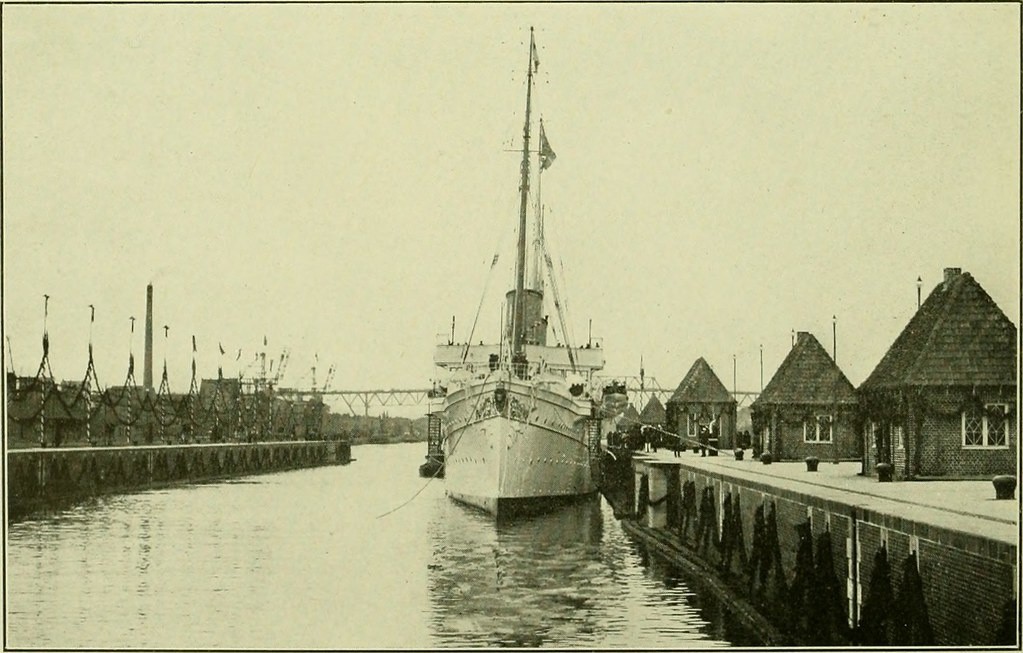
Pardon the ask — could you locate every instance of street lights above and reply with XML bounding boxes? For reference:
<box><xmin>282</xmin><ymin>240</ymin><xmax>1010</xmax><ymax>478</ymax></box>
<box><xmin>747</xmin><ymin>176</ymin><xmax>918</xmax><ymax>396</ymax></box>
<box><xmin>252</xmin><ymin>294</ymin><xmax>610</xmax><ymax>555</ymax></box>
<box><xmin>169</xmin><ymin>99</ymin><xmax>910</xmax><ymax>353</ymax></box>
<box><xmin>917</xmin><ymin>275</ymin><xmax>923</xmax><ymax>309</ymax></box>
<box><xmin>832</xmin><ymin>314</ymin><xmax>837</xmax><ymax>363</ymax></box>
<box><xmin>759</xmin><ymin>344</ymin><xmax>764</xmax><ymax>392</ymax></box>
<box><xmin>733</xmin><ymin>354</ymin><xmax>737</xmax><ymax>400</ymax></box>
<box><xmin>791</xmin><ymin>328</ymin><xmax>795</xmax><ymax>347</ymax></box>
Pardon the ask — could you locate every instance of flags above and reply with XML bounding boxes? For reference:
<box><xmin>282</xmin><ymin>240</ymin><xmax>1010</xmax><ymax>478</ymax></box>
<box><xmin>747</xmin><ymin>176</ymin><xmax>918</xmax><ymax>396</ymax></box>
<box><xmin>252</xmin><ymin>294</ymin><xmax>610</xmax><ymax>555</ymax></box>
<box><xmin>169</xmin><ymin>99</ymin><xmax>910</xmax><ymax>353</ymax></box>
<box><xmin>540</xmin><ymin>125</ymin><xmax>557</xmax><ymax>173</ymax></box>
<box><xmin>532</xmin><ymin>33</ymin><xmax>540</xmax><ymax>72</ymax></box>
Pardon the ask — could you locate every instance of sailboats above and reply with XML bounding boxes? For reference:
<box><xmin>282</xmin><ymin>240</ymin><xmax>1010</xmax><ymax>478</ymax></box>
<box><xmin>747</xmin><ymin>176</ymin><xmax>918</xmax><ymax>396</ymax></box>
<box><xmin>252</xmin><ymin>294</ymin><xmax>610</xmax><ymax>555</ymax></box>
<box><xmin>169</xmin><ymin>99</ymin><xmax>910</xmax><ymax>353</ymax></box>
<box><xmin>431</xmin><ymin>25</ymin><xmax>604</xmax><ymax>521</ymax></box>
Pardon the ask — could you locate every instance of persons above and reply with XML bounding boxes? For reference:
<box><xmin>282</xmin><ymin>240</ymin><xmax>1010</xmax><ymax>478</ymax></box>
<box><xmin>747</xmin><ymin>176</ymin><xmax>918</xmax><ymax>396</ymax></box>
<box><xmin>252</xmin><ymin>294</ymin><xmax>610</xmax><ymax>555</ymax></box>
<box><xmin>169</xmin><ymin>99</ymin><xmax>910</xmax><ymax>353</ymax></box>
<box><xmin>608</xmin><ymin>423</ymin><xmax>710</xmax><ymax>458</ymax></box>
